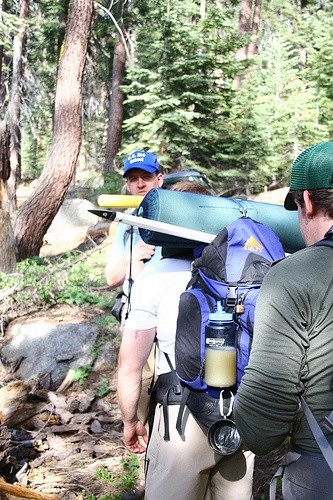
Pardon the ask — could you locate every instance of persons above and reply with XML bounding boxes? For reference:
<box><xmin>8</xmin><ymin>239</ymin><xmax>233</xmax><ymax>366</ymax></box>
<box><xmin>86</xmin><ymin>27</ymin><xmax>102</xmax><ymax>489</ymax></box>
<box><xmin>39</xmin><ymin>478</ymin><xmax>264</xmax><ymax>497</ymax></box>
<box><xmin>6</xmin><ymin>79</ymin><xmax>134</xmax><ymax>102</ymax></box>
<box><xmin>234</xmin><ymin>141</ymin><xmax>332</xmax><ymax>500</ymax></box>
<box><xmin>105</xmin><ymin>151</ymin><xmax>165</xmax><ymax>286</ymax></box>
<box><xmin>116</xmin><ymin>181</ymin><xmax>255</xmax><ymax>500</ymax></box>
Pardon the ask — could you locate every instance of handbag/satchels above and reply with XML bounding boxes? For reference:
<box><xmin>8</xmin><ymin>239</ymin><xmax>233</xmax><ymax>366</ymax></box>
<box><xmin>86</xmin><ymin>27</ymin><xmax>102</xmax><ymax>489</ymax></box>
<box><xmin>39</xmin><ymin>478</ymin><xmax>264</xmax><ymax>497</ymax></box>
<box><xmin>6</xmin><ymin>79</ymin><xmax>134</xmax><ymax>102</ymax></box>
<box><xmin>111</xmin><ymin>291</ymin><xmax>128</xmax><ymax>321</ymax></box>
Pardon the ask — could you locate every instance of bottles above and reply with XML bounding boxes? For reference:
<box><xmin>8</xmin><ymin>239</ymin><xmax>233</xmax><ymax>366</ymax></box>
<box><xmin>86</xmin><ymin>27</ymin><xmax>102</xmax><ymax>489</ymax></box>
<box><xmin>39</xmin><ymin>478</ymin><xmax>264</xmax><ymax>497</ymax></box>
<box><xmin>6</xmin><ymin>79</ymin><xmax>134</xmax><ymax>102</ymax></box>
<box><xmin>205</xmin><ymin>301</ymin><xmax>237</xmax><ymax>387</ymax></box>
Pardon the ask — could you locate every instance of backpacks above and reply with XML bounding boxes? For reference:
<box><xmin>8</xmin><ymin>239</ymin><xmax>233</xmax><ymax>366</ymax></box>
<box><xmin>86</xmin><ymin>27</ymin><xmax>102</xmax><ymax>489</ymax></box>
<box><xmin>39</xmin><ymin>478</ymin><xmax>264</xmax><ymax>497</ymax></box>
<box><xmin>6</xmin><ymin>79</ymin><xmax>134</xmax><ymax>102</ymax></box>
<box><xmin>145</xmin><ymin>216</ymin><xmax>285</xmax><ymax>441</ymax></box>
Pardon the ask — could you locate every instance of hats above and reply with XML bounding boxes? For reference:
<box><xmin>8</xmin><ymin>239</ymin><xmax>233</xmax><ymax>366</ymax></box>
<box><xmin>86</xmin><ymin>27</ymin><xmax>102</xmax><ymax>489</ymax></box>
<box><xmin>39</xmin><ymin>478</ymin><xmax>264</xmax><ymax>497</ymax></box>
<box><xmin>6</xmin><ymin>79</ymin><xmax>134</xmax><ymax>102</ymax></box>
<box><xmin>284</xmin><ymin>138</ymin><xmax>333</xmax><ymax>211</ymax></box>
<box><xmin>122</xmin><ymin>151</ymin><xmax>161</xmax><ymax>177</ymax></box>
